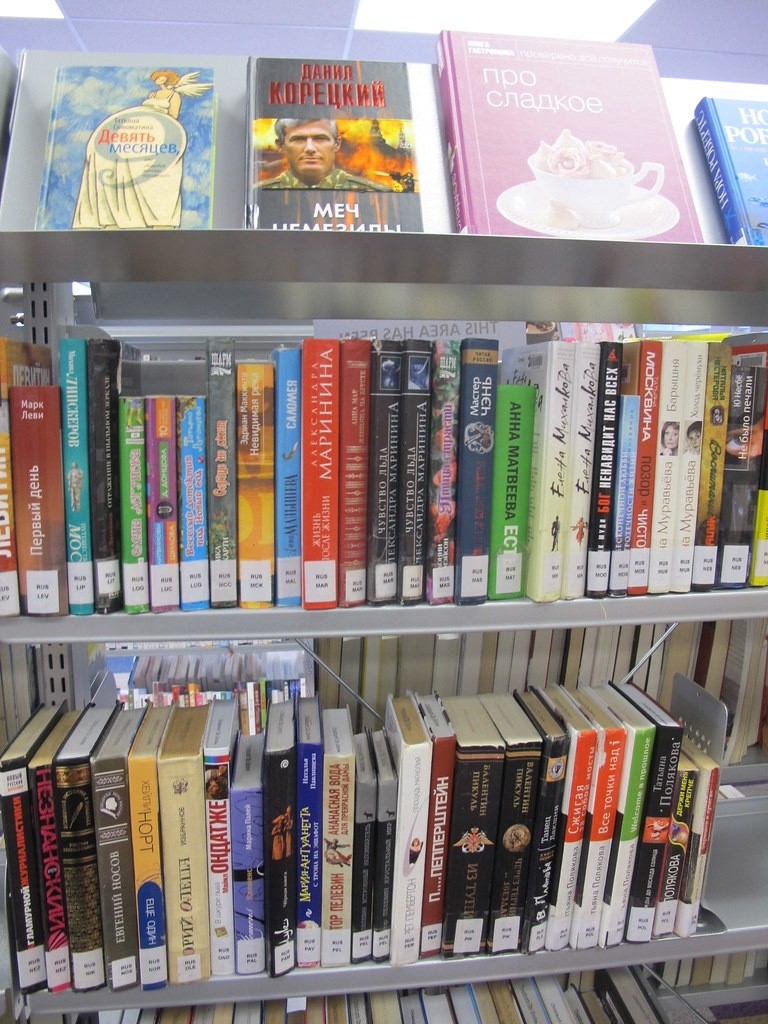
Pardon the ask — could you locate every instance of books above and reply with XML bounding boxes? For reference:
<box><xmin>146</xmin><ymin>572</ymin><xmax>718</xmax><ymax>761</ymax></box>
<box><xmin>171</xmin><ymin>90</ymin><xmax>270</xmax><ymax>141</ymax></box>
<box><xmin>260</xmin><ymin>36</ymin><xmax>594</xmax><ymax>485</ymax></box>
<box><xmin>0</xmin><ymin>616</ymin><xmax>768</xmax><ymax>1024</ymax></box>
<box><xmin>0</xmin><ymin>30</ymin><xmax>706</xmax><ymax>244</ymax></box>
<box><xmin>694</xmin><ymin>96</ymin><xmax>768</xmax><ymax>247</ymax></box>
<box><xmin>0</xmin><ymin>337</ymin><xmax>768</xmax><ymax>620</ymax></box>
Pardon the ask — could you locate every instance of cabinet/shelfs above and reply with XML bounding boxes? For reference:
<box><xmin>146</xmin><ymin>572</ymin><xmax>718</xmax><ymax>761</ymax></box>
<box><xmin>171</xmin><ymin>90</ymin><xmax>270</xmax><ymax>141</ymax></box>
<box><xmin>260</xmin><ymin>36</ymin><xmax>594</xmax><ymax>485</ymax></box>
<box><xmin>2</xmin><ymin>50</ymin><xmax>767</xmax><ymax>1023</ymax></box>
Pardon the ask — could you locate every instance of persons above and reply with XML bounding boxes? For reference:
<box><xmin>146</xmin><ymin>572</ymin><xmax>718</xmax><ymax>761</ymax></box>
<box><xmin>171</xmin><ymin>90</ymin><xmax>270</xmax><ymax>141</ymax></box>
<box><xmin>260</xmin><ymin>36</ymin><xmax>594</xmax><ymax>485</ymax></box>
<box><xmin>255</xmin><ymin>116</ymin><xmax>394</xmax><ymax>193</ymax></box>
<box><xmin>660</xmin><ymin>421</ymin><xmax>678</xmax><ymax>455</ymax></box>
<box><xmin>685</xmin><ymin>421</ymin><xmax>702</xmax><ymax>455</ymax></box>
<box><xmin>724</xmin><ymin>364</ymin><xmax>768</xmax><ymax>467</ymax></box>
